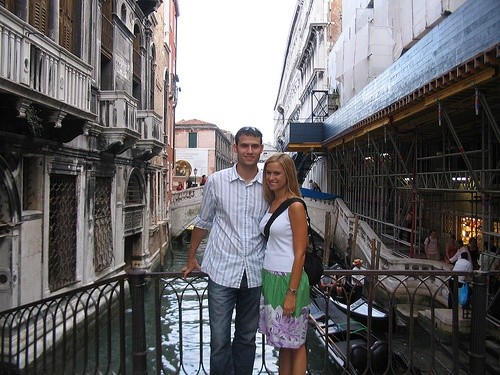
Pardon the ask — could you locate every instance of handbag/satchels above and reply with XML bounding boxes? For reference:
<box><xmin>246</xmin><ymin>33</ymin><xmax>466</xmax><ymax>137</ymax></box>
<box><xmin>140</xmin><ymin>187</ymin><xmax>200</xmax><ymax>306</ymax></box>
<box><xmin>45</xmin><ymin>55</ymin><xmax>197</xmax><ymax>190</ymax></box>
<box><xmin>304</xmin><ymin>252</ymin><xmax>324</xmax><ymax>285</ymax></box>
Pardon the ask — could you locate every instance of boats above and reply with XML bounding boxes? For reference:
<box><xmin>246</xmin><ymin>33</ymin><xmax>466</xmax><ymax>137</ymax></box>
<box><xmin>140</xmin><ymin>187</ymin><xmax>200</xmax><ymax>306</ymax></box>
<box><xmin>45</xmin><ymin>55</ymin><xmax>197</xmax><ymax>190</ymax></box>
<box><xmin>308</xmin><ymin>288</ymin><xmax>423</xmax><ymax>375</ymax></box>
<box><xmin>313</xmin><ymin>275</ymin><xmax>389</xmax><ymax>319</ymax></box>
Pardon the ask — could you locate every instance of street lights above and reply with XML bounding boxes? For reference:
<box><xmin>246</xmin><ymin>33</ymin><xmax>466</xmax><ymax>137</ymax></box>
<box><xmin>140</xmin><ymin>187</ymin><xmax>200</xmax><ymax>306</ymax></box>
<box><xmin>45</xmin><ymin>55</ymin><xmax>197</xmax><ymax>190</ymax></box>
<box><xmin>194</xmin><ymin>168</ymin><xmax>197</xmax><ymax>185</ymax></box>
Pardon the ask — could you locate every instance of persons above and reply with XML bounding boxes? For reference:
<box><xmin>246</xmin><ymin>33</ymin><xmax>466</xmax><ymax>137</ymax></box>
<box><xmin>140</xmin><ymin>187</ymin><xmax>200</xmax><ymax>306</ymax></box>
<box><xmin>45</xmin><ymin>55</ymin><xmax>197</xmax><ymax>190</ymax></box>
<box><xmin>331</xmin><ymin>259</ymin><xmax>368</xmax><ymax>305</ymax></box>
<box><xmin>200</xmin><ymin>175</ymin><xmax>207</xmax><ymax>186</ymax></box>
<box><xmin>424</xmin><ymin>230</ymin><xmax>441</xmax><ymax>260</ymax></box>
<box><xmin>258</xmin><ymin>153</ymin><xmax>325</xmax><ymax>375</ymax></box>
<box><xmin>186</xmin><ymin>177</ymin><xmax>193</xmax><ymax>189</ymax></box>
<box><xmin>445</xmin><ymin>234</ymin><xmax>480</xmax><ymax>310</ymax></box>
<box><xmin>176</xmin><ymin>181</ymin><xmax>184</xmax><ymax>191</ymax></box>
<box><xmin>181</xmin><ymin>127</ymin><xmax>269</xmax><ymax>375</ymax></box>
<box><xmin>309</xmin><ymin>180</ymin><xmax>321</xmax><ymax>192</ymax></box>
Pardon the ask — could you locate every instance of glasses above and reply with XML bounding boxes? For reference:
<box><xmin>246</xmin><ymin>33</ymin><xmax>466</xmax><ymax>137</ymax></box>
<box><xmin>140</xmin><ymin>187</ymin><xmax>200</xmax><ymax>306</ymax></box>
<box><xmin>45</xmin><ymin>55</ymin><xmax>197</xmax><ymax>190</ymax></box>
<box><xmin>237</xmin><ymin>126</ymin><xmax>262</xmax><ymax>137</ymax></box>
